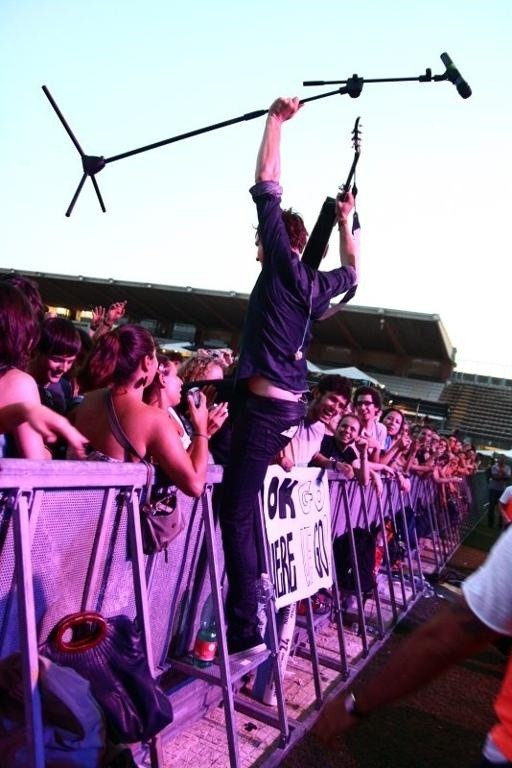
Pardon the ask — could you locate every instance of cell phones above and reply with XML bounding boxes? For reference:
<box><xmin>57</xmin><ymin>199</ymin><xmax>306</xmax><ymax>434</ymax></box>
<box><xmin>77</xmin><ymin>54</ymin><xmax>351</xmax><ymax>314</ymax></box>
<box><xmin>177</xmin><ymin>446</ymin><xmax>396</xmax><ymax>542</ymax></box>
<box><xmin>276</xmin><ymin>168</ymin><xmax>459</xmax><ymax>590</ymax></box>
<box><xmin>191</xmin><ymin>387</ymin><xmax>200</xmax><ymax>408</ymax></box>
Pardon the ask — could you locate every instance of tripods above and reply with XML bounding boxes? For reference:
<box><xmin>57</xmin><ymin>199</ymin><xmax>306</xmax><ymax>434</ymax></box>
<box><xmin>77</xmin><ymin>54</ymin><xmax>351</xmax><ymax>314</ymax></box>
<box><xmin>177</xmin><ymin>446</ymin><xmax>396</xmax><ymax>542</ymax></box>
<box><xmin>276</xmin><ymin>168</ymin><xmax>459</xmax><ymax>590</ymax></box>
<box><xmin>41</xmin><ymin>73</ymin><xmax>441</xmax><ymax>218</ymax></box>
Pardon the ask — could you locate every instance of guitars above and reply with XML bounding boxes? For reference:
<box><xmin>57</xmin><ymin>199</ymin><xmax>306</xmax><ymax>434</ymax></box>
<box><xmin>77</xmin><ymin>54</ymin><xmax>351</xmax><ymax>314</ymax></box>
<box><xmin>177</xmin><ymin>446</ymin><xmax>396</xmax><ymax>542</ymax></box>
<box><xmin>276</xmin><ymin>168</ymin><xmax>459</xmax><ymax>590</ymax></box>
<box><xmin>299</xmin><ymin>117</ymin><xmax>362</xmax><ymax>269</ymax></box>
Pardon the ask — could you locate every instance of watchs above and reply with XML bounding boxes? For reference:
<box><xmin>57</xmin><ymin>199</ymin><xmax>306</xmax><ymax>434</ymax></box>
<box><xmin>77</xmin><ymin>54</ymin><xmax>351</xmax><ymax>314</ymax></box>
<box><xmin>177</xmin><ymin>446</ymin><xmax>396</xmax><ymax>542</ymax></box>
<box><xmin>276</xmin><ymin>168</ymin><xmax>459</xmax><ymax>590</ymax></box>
<box><xmin>344</xmin><ymin>691</ymin><xmax>372</xmax><ymax>722</ymax></box>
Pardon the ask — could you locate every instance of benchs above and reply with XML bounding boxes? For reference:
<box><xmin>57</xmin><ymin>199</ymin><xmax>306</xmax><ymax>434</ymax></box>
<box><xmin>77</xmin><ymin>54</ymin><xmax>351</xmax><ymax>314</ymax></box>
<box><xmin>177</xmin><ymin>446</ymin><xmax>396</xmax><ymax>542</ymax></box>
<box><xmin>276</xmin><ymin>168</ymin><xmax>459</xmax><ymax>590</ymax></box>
<box><xmin>365</xmin><ymin>372</ymin><xmax>512</xmax><ymax>450</ymax></box>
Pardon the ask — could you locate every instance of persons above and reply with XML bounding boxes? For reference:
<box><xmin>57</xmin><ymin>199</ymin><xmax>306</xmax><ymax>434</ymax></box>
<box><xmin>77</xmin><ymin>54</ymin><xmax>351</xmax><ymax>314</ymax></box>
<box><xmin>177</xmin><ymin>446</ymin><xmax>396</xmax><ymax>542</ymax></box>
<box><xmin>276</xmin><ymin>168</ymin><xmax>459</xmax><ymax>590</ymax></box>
<box><xmin>310</xmin><ymin>455</ymin><xmax>511</xmax><ymax>768</ymax></box>
<box><xmin>216</xmin><ymin>96</ymin><xmax>361</xmax><ymax>664</ymax></box>
<box><xmin>463</xmin><ymin>456</ymin><xmax>512</xmax><ymax>567</ymax></box>
<box><xmin>2</xmin><ymin>271</ymin><xmax>484</xmax><ymax>610</ymax></box>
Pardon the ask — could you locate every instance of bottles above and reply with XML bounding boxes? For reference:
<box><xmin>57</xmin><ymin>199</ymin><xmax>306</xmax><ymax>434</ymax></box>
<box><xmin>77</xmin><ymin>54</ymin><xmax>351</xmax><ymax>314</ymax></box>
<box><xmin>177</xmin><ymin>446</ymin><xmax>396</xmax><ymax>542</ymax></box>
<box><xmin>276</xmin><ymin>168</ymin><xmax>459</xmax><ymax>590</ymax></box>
<box><xmin>255</xmin><ymin>573</ymin><xmax>274</xmax><ymax>640</ymax></box>
<box><xmin>193</xmin><ymin>621</ymin><xmax>218</xmax><ymax>668</ymax></box>
<box><xmin>408</xmin><ymin>576</ymin><xmax>434</xmax><ymax>598</ymax></box>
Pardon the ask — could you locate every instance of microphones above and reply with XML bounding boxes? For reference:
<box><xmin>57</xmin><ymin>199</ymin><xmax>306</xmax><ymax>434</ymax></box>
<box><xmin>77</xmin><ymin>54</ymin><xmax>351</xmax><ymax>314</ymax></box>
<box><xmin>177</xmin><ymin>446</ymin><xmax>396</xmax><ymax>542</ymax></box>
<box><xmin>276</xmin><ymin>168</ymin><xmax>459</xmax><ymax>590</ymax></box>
<box><xmin>440</xmin><ymin>52</ymin><xmax>472</xmax><ymax>100</ymax></box>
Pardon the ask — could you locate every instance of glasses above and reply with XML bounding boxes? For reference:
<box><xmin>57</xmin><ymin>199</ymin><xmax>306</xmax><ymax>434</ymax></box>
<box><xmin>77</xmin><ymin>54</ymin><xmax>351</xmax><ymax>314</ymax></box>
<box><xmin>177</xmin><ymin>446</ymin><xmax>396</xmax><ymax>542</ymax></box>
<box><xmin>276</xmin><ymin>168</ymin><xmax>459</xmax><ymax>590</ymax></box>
<box><xmin>357</xmin><ymin>401</ymin><xmax>374</xmax><ymax>406</ymax></box>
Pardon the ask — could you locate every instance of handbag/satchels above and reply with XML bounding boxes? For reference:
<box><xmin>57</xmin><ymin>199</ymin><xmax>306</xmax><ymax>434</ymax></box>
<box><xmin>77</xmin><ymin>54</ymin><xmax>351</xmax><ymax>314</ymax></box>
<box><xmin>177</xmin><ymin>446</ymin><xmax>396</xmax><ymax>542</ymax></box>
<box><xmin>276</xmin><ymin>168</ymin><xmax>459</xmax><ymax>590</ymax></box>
<box><xmin>140</xmin><ymin>491</ymin><xmax>188</xmax><ymax>563</ymax></box>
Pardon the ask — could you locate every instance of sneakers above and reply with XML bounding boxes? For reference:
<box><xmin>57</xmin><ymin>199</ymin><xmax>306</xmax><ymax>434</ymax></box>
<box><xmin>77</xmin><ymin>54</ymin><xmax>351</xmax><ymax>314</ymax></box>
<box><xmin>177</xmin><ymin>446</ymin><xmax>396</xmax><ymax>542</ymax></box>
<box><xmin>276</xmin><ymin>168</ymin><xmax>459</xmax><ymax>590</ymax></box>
<box><xmin>215</xmin><ymin>633</ymin><xmax>267</xmax><ymax>665</ymax></box>
